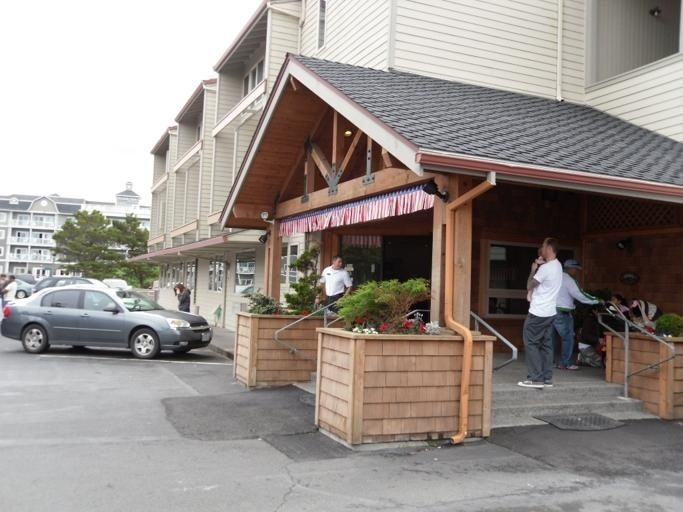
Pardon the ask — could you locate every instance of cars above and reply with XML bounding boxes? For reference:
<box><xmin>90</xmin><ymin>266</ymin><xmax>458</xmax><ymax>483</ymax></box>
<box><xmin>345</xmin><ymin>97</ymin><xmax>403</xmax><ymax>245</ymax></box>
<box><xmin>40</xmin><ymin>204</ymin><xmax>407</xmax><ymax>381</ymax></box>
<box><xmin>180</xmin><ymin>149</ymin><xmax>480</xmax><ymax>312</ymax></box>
<box><xmin>1</xmin><ymin>273</ymin><xmax>131</xmax><ymax>301</ymax></box>
<box><xmin>0</xmin><ymin>285</ymin><xmax>212</xmax><ymax>358</ymax></box>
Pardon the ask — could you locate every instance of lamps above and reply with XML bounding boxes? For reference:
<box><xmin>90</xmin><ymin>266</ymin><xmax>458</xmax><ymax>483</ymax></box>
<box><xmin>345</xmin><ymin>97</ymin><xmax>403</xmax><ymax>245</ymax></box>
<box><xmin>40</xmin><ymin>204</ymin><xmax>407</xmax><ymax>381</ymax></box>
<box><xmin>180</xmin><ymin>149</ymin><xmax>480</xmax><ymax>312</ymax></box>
<box><xmin>344</xmin><ymin>128</ymin><xmax>353</xmax><ymax>138</ymax></box>
<box><xmin>542</xmin><ymin>188</ymin><xmax>559</xmax><ymax>208</ymax></box>
<box><xmin>260</xmin><ymin>230</ymin><xmax>270</xmax><ymax>244</ymax></box>
<box><xmin>622</xmin><ymin>272</ymin><xmax>641</xmax><ymax>300</ymax></box>
<box><xmin>618</xmin><ymin>239</ymin><xmax>632</xmax><ymax>249</ymax></box>
<box><xmin>260</xmin><ymin>210</ymin><xmax>275</xmax><ymax>227</ymax></box>
<box><xmin>421</xmin><ymin>180</ymin><xmax>448</xmax><ymax>203</ymax></box>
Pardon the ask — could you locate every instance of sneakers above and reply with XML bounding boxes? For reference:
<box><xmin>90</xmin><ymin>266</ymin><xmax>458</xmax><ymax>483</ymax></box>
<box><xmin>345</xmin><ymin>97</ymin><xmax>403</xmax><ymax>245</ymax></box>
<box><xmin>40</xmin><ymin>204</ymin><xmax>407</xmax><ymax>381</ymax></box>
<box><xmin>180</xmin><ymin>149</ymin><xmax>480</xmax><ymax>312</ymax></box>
<box><xmin>558</xmin><ymin>362</ymin><xmax>578</xmax><ymax>370</ymax></box>
<box><xmin>518</xmin><ymin>381</ymin><xmax>553</xmax><ymax>388</ymax></box>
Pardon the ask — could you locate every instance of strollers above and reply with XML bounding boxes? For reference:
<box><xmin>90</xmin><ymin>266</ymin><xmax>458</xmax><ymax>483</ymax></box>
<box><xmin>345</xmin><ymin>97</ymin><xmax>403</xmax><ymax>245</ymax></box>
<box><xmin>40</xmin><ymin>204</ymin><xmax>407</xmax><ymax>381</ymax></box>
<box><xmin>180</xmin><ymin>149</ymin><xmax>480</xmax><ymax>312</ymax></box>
<box><xmin>599</xmin><ymin>298</ymin><xmax>664</xmax><ymax>365</ymax></box>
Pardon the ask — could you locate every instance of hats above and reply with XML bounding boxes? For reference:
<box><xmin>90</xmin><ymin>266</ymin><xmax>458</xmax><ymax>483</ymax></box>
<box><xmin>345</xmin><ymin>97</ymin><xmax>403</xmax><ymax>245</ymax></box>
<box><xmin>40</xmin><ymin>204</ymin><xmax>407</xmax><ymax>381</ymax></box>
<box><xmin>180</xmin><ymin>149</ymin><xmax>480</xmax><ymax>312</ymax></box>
<box><xmin>564</xmin><ymin>259</ymin><xmax>582</xmax><ymax>270</ymax></box>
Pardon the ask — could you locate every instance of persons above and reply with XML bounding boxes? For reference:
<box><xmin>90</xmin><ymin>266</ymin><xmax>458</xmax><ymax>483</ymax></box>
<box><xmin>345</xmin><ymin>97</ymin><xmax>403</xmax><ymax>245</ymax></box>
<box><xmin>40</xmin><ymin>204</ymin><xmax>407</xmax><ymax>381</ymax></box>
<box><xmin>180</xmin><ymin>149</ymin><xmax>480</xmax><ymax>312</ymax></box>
<box><xmin>525</xmin><ymin>244</ymin><xmax>547</xmax><ymax>302</ymax></box>
<box><xmin>572</xmin><ymin>293</ymin><xmax>663</xmax><ymax>369</ymax></box>
<box><xmin>173</xmin><ymin>282</ymin><xmax>191</xmax><ymax>313</ymax></box>
<box><xmin>550</xmin><ymin>258</ymin><xmax>606</xmax><ymax>371</ymax></box>
<box><xmin>0</xmin><ymin>273</ymin><xmax>7</xmax><ymax>296</ymax></box>
<box><xmin>516</xmin><ymin>235</ymin><xmax>564</xmax><ymax>390</ymax></box>
<box><xmin>0</xmin><ymin>274</ymin><xmax>18</xmax><ymax>308</ymax></box>
<box><xmin>314</xmin><ymin>255</ymin><xmax>353</xmax><ymax>315</ymax></box>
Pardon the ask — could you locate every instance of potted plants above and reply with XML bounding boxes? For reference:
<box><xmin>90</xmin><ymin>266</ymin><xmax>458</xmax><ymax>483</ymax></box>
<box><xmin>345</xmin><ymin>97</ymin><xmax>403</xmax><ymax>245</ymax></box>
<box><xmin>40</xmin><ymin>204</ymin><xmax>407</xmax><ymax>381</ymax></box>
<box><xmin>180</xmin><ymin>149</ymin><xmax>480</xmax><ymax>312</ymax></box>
<box><xmin>318</xmin><ymin>278</ymin><xmax>495</xmax><ymax>447</ymax></box>
<box><xmin>234</xmin><ymin>243</ymin><xmax>351</xmax><ymax>388</ymax></box>
<box><xmin>606</xmin><ymin>313</ymin><xmax>683</xmax><ymax>420</ymax></box>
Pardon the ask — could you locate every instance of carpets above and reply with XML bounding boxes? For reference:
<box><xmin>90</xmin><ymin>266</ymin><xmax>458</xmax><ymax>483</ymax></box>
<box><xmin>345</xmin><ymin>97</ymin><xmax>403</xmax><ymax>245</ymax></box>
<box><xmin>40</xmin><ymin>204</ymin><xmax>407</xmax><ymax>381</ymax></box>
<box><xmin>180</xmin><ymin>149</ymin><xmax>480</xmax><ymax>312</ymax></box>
<box><xmin>532</xmin><ymin>411</ymin><xmax>629</xmax><ymax>431</ymax></box>
<box><xmin>161</xmin><ymin>384</ymin><xmax>318</xmax><ymax>441</ymax></box>
